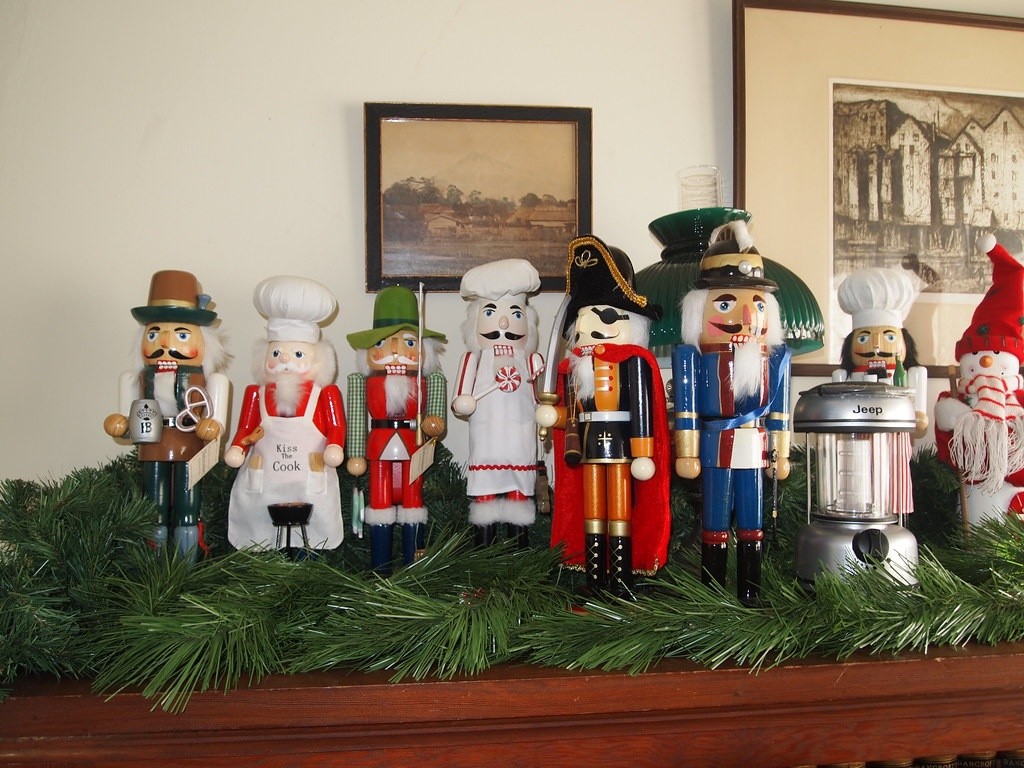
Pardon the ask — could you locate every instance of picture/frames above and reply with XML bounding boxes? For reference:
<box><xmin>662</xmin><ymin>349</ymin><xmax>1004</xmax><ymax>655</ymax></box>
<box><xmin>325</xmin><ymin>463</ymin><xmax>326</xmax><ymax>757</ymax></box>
<box><xmin>364</xmin><ymin>102</ymin><xmax>594</xmax><ymax>294</ymax></box>
<box><xmin>732</xmin><ymin>0</ymin><xmax>1024</xmax><ymax>378</ymax></box>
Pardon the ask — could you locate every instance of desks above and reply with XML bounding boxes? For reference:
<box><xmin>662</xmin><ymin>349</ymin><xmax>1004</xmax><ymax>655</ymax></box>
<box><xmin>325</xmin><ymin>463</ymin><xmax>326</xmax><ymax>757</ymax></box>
<box><xmin>0</xmin><ymin>639</ymin><xmax>1024</xmax><ymax>768</ymax></box>
